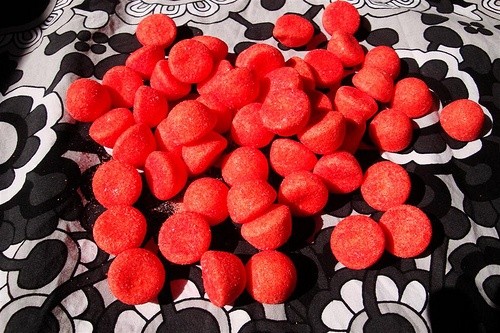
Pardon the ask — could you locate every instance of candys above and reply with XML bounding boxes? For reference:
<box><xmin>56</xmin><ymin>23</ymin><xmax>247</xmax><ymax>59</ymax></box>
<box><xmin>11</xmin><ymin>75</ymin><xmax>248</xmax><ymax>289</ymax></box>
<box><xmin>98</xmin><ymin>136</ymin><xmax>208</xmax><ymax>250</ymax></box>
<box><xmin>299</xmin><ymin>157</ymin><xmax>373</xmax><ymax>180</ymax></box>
<box><xmin>64</xmin><ymin>0</ymin><xmax>484</xmax><ymax>310</ymax></box>
